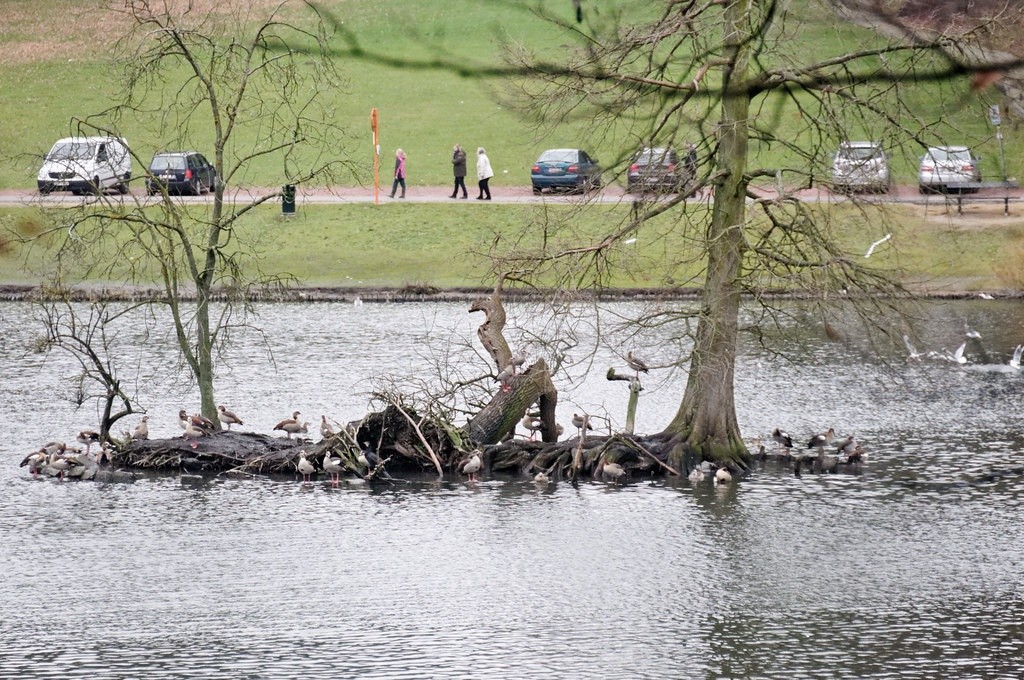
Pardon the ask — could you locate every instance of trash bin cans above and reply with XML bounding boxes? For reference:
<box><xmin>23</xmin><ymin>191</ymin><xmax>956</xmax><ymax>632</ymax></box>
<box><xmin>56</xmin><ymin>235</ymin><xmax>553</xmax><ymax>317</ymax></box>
<box><xmin>281</xmin><ymin>185</ymin><xmax>296</xmax><ymax>216</ymax></box>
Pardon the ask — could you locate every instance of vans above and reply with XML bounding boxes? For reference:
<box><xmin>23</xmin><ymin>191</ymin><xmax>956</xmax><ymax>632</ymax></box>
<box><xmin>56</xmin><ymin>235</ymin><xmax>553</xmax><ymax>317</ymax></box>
<box><xmin>37</xmin><ymin>137</ymin><xmax>131</xmax><ymax>197</ymax></box>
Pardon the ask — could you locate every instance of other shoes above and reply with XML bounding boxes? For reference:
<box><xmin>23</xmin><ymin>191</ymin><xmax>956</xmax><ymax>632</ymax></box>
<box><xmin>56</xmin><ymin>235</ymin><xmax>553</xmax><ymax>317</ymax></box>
<box><xmin>476</xmin><ymin>196</ymin><xmax>491</xmax><ymax>200</ymax></box>
<box><xmin>460</xmin><ymin>197</ymin><xmax>467</xmax><ymax>199</ymax></box>
<box><xmin>449</xmin><ymin>196</ymin><xmax>456</xmax><ymax>199</ymax></box>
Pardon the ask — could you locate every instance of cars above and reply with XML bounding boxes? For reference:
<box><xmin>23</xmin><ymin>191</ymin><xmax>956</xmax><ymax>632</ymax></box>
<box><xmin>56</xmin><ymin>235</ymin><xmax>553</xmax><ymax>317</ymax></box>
<box><xmin>627</xmin><ymin>148</ymin><xmax>680</xmax><ymax>193</ymax></box>
<box><xmin>917</xmin><ymin>147</ymin><xmax>982</xmax><ymax>194</ymax></box>
<box><xmin>531</xmin><ymin>149</ymin><xmax>601</xmax><ymax>195</ymax></box>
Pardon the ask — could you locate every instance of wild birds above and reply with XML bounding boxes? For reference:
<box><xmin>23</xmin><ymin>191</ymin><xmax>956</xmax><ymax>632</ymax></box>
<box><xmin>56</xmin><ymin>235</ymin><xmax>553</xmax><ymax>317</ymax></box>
<box><xmin>20</xmin><ymin>405</ymin><xmax>216</xmax><ymax>486</ymax></box>
<box><xmin>218</xmin><ymin>405</ymin><xmax>244</xmax><ymax>434</ymax></box>
<box><xmin>863</xmin><ymin>232</ymin><xmax>1024</xmax><ymax>371</ymax></box>
<box><xmin>273</xmin><ymin>410</ymin><xmax>385</xmax><ymax>488</ymax></box>
<box><xmin>494</xmin><ymin>352</ymin><xmax>870</xmax><ymax>486</ymax></box>
<box><xmin>457</xmin><ymin>450</ymin><xmax>483</xmax><ymax>484</ymax></box>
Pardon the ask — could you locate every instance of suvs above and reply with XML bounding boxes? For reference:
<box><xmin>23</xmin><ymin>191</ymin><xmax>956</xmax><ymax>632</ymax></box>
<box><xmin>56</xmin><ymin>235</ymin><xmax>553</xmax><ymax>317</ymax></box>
<box><xmin>832</xmin><ymin>139</ymin><xmax>891</xmax><ymax>193</ymax></box>
<box><xmin>145</xmin><ymin>150</ymin><xmax>216</xmax><ymax>196</ymax></box>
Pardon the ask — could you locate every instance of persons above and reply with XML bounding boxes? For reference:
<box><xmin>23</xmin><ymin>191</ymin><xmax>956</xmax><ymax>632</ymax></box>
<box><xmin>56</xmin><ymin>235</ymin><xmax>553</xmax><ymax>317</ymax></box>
<box><xmin>387</xmin><ymin>148</ymin><xmax>406</xmax><ymax>198</ymax></box>
<box><xmin>681</xmin><ymin>140</ymin><xmax>697</xmax><ymax>198</ymax></box>
<box><xmin>669</xmin><ymin>143</ymin><xmax>681</xmax><ymax>193</ymax></box>
<box><xmin>448</xmin><ymin>144</ymin><xmax>468</xmax><ymax>199</ymax></box>
<box><xmin>475</xmin><ymin>147</ymin><xmax>493</xmax><ymax>200</ymax></box>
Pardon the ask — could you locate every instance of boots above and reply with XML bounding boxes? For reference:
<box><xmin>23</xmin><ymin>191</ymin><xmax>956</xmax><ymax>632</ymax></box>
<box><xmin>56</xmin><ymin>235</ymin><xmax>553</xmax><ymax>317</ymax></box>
<box><xmin>384</xmin><ymin>190</ymin><xmax>396</xmax><ymax>198</ymax></box>
<box><xmin>399</xmin><ymin>192</ymin><xmax>405</xmax><ymax>198</ymax></box>
